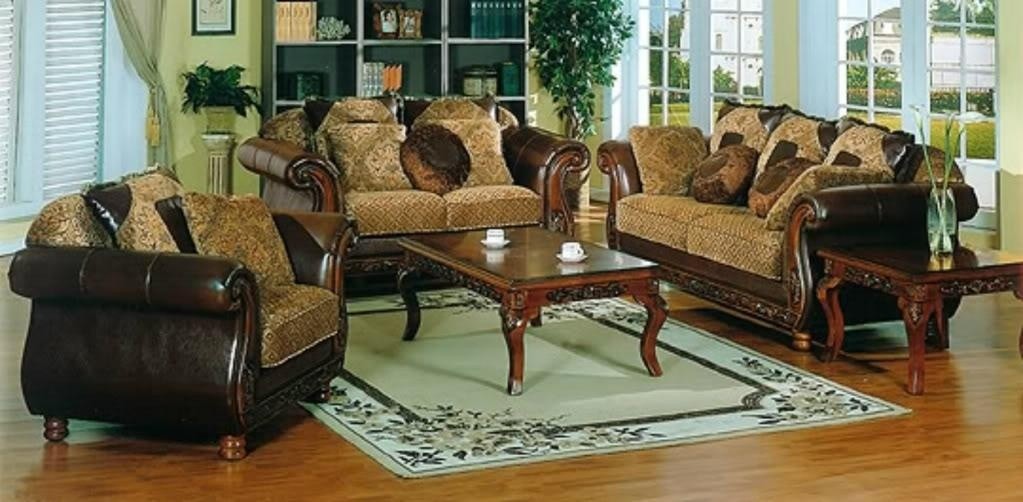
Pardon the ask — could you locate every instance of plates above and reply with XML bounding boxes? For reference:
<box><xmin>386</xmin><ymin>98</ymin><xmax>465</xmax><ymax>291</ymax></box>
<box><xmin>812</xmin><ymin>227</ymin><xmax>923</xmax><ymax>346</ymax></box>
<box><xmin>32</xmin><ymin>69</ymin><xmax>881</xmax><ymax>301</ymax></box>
<box><xmin>480</xmin><ymin>240</ymin><xmax>510</xmax><ymax>248</ymax></box>
<box><xmin>556</xmin><ymin>254</ymin><xmax>587</xmax><ymax>262</ymax></box>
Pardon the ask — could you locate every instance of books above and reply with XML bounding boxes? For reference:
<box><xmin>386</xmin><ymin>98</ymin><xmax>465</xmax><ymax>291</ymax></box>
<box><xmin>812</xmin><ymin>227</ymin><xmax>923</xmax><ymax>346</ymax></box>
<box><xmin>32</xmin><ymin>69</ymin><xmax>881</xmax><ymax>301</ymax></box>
<box><xmin>362</xmin><ymin>60</ymin><xmax>385</xmax><ymax>96</ymax></box>
<box><xmin>277</xmin><ymin>2</ymin><xmax>317</xmax><ymax>43</ymax></box>
<box><xmin>469</xmin><ymin>0</ymin><xmax>525</xmax><ymax>38</ymax></box>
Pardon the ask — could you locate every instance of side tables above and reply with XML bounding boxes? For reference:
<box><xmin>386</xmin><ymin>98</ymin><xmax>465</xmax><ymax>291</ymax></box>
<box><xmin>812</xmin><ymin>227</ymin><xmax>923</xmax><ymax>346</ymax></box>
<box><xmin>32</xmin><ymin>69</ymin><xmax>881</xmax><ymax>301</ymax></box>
<box><xmin>814</xmin><ymin>236</ymin><xmax>1023</xmax><ymax>407</ymax></box>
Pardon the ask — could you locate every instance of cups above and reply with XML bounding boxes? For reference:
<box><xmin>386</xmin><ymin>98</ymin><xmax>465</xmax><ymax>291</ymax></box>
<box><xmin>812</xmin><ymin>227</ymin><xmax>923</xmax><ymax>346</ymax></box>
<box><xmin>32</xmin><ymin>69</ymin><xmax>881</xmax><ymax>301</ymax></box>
<box><xmin>562</xmin><ymin>242</ymin><xmax>583</xmax><ymax>258</ymax></box>
<box><xmin>486</xmin><ymin>229</ymin><xmax>504</xmax><ymax>242</ymax></box>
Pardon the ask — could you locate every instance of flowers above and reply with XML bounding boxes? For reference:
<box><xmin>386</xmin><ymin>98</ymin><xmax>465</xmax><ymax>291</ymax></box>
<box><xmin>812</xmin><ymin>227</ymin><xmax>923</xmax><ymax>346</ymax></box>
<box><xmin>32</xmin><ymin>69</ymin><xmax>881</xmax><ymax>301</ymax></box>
<box><xmin>901</xmin><ymin>102</ymin><xmax>988</xmax><ymax>253</ymax></box>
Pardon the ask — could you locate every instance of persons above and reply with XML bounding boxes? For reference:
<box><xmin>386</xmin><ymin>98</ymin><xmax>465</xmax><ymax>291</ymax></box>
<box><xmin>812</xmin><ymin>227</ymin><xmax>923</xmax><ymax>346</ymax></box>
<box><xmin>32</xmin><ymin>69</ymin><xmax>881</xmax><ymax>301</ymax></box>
<box><xmin>382</xmin><ymin>10</ymin><xmax>395</xmax><ymax>32</ymax></box>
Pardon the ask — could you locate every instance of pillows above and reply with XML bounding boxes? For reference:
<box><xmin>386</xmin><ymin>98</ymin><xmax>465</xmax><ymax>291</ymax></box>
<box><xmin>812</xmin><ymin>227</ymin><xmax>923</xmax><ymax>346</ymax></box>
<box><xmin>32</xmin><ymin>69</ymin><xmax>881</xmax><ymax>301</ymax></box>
<box><xmin>709</xmin><ymin>97</ymin><xmax>782</xmax><ymax>157</ymax></box>
<box><xmin>399</xmin><ymin>124</ymin><xmax>471</xmax><ymax>193</ymax></box>
<box><xmin>413</xmin><ymin>117</ymin><xmax>515</xmax><ymax>186</ymax></box>
<box><xmin>403</xmin><ymin>89</ymin><xmax>497</xmax><ymax>134</ymax></box>
<box><xmin>764</xmin><ymin>166</ymin><xmax>894</xmax><ymax>229</ymax></box>
<box><xmin>182</xmin><ymin>190</ymin><xmax>294</xmax><ymax>291</ymax></box>
<box><xmin>692</xmin><ymin>143</ymin><xmax>758</xmax><ymax>204</ymax></box>
<box><xmin>822</xmin><ymin>116</ymin><xmax>915</xmax><ymax>174</ymax></box>
<box><xmin>627</xmin><ymin>125</ymin><xmax>708</xmax><ymax>196</ymax></box>
<box><xmin>753</xmin><ymin>104</ymin><xmax>839</xmax><ymax>180</ymax></box>
<box><xmin>326</xmin><ymin>123</ymin><xmax>414</xmax><ymax>193</ymax></box>
<box><xmin>747</xmin><ymin>157</ymin><xmax>814</xmax><ymax>218</ymax></box>
<box><xmin>303</xmin><ymin>94</ymin><xmax>398</xmax><ymax>160</ymax></box>
<box><xmin>80</xmin><ymin>161</ymin><xmax>197</xmax><ymax>253</ymax></box>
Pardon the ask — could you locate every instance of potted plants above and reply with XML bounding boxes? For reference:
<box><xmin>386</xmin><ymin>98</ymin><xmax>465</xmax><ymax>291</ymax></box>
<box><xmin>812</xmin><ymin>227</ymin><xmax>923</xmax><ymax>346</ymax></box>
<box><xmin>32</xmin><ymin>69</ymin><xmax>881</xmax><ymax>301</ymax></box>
<box><xmin>180</xmin><ymin>61</ymin><xmax>261</xmax><ymax>135</ymax></box>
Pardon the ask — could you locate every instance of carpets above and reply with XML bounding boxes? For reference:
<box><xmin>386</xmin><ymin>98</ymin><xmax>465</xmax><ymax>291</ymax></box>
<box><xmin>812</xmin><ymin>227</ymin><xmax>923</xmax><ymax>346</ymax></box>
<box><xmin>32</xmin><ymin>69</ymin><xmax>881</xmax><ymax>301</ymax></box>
<box><xmin>298</xmin><ymin>282</ymin><xmax>912</xmax><ymax>481</ymax></box>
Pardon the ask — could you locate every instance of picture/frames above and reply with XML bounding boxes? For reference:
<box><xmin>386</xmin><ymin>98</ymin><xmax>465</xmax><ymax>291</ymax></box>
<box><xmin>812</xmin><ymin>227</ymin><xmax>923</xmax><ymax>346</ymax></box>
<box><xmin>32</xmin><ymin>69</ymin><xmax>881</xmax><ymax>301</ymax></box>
<box><xmin>398</xmin><ymin>9</ymin><xmax>422</xmax><ymax>39</ymax></box>
<box><xmin>191</xmin><ymin>0</ymin><xmax>235</xmax><ymax>37</ymax></box>
<box><xmin>370</xmin><ymin>1</ymin><xmax>402</xmax><ymax>38</ymax></box>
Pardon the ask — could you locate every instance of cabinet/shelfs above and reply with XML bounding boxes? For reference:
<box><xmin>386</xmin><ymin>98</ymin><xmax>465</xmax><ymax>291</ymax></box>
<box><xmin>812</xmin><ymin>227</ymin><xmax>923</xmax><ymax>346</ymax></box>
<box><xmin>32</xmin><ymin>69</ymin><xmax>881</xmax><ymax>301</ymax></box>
<box><xmin>445</xmin><ymin>0</ymin><xmax>530</xmax><ymax>124</ymax></box>
<box><xmin>260</xmin><ymin>0</ymin><xmax>360</xmax><ymax>133</ymax></box>
<box><xmin>360</xmin><ymin>0</ymin><xmax>445</xmax><ymax>98</ymax></box>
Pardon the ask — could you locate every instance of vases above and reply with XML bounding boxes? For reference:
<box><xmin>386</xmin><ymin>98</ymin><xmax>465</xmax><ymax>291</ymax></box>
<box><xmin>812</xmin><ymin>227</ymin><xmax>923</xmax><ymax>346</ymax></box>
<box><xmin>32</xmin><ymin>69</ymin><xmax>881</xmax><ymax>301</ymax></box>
<box><xmin>925</xmin><ymin>187</ymin><xmax>957</xmax><ymax>252</ymax></box>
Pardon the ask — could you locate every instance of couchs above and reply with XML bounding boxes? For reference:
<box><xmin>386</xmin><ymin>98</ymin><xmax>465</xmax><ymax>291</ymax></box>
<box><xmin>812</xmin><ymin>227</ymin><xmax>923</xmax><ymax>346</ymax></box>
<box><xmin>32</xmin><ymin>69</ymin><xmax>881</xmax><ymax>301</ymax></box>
<box><xmin>10</xmin><ymin>165</ymin><xmax>359</xmax><ymax>461</ymax></box>
<box><xmin>237</xmin><ymin>89</ymin><xmax>590</xmax><ymax>297</ymax></box>
<box><xmin>595</xmin><ymin>97</ymin><xmax>979</xmax><ymax>352</ymax></box>
